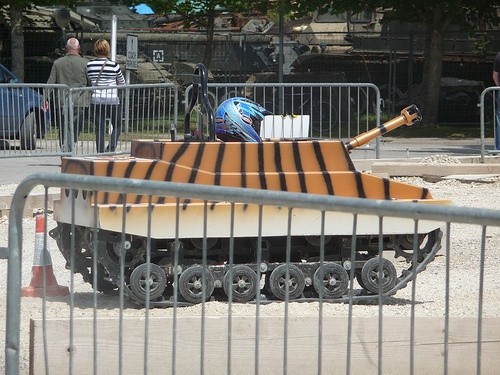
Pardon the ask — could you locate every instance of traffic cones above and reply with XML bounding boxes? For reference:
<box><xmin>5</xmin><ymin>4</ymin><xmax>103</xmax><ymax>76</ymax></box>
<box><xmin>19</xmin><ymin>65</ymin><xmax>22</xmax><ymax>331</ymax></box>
<box><xmin>20</xmin><ymin>212</ymin><xmax>69</xmax><ymax>298</ymax></box>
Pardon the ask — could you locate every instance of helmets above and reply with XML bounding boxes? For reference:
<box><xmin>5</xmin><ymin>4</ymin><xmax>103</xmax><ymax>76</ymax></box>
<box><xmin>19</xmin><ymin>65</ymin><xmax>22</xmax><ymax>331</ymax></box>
<box><xmin>215</xmin><ymin>96</ymin><xmax>273</xmax><ymax>142</ymax></box>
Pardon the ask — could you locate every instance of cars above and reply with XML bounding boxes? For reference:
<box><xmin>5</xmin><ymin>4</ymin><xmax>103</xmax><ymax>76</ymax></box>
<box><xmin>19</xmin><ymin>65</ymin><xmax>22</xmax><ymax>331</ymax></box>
<box><xmin>0</xmin><ymin>64</ymin><xmax>50</xmax><ymax>150</ymax></box>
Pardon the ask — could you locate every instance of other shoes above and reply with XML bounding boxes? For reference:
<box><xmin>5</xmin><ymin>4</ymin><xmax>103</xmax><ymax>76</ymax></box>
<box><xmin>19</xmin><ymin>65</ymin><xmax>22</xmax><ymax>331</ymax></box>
<box><xmin>106</xmin><ymin>145</ymin><xmax>114</xmax><ymax>153</ymax></box>
<box><xmin>60</xmin><ymin>145</ymin><xmax>70</xmax><ymax>158</ymax></box>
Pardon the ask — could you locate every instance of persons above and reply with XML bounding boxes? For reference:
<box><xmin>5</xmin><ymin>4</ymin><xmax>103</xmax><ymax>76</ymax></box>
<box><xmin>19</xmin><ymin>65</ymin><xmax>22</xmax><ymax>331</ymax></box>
<box><xmin>492</xmin><ymin>51</ymin><xmax>500</xmax><ymax>150</ymax></box>
<box><xmin>214</xmin><ymin>97</ymin><xmax>275</xmax><ymax>142</ymax></box>
<box><xmin>86</xmin><ymin>38</ymin><xmax>126</xmax><ymax>154</ymax></box>
<box><xmin>41</xmin><ymin>38</ymin><xmax>91</xmax><ymax>158</ymax></box>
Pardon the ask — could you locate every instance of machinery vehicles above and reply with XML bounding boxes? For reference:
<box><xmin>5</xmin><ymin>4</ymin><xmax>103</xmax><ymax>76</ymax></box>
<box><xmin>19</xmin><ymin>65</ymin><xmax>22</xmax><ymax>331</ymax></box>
<box><xmin>49</xmin><ymin>103</ymin><xmax>443</xmax><ymax>310</ymax></box>
